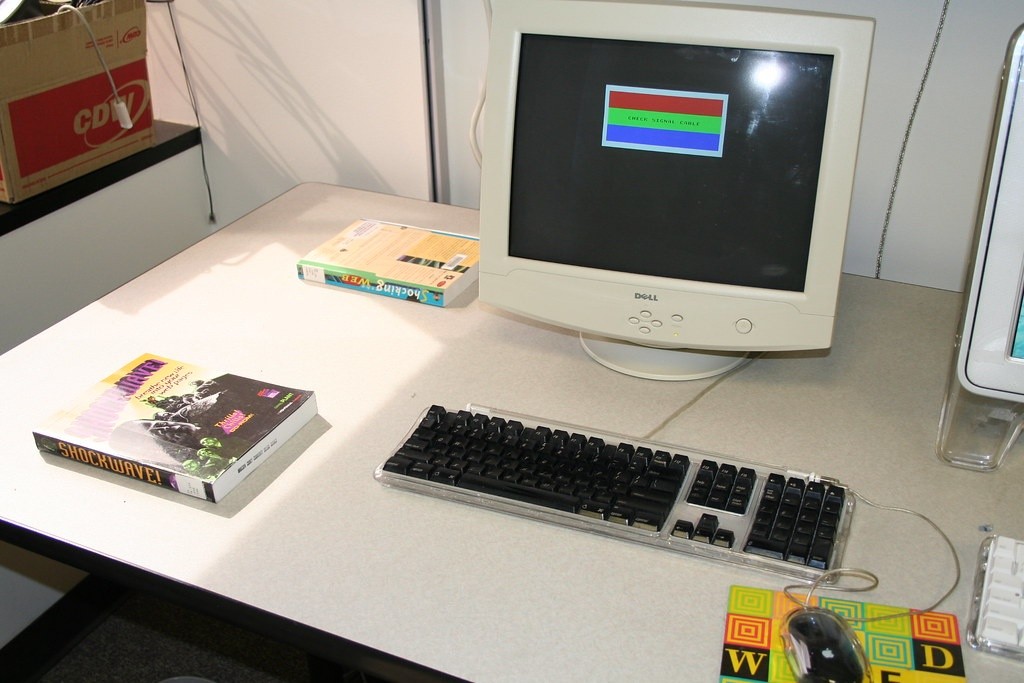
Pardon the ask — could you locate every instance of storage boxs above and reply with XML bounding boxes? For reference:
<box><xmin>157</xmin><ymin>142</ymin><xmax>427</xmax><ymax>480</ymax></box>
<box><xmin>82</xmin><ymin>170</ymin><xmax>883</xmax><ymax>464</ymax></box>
<box><xmin>0</xmin><ymin>0</ymin><xmax>155</xmax><ymax>204</ymax></box>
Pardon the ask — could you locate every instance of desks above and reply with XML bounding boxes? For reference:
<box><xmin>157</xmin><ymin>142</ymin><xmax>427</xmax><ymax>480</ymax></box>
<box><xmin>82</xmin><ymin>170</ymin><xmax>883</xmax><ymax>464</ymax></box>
<box><xmin>0</xmin><ymin>181</ymin><xmax>1024</xmax><ymax>683</ymax></box>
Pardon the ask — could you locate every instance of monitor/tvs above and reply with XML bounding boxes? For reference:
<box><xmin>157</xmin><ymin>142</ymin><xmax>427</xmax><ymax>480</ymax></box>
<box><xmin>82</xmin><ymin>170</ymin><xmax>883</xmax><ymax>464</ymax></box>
<box><xmin>935</xmin><ymin>24</ymin><xmax>1024</xmax><ymax>471</ymax></box>
<box><xmin>479</xmin><ymin>0</ymin><xmax>870</xmax><ymax>384</ymax></box>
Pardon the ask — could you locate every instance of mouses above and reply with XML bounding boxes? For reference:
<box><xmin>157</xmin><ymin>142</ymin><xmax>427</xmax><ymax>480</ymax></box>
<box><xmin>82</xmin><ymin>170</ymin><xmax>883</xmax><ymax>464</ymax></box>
<box><xmin>779</xmin><ymin>606</ymin><xmax>873</xmax><ymax>683</ymax></box>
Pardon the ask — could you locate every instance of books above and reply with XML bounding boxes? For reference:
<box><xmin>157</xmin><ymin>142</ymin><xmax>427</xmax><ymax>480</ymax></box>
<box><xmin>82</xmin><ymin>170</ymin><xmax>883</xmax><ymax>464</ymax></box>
<box><xmin>33</xmin><ymin>352</ymin><xmax>319</xmax><ymax>504</ymax></box>
<box><xmin>298</xmin><ymin>218</ymin><xmax>479</xmax><ymax>308</ymax></box>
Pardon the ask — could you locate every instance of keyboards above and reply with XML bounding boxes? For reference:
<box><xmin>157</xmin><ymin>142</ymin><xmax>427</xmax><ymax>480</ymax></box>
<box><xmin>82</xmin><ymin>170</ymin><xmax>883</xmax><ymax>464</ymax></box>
<box><xmin>373</xmin><ymin>405</ymin><xmax>855</xmax><ymax>585</ymax></box>
<box><xmin>966</xmin><ymin>535</ymin><xmax>1024</xmax><ymax>662</ymax></box>
